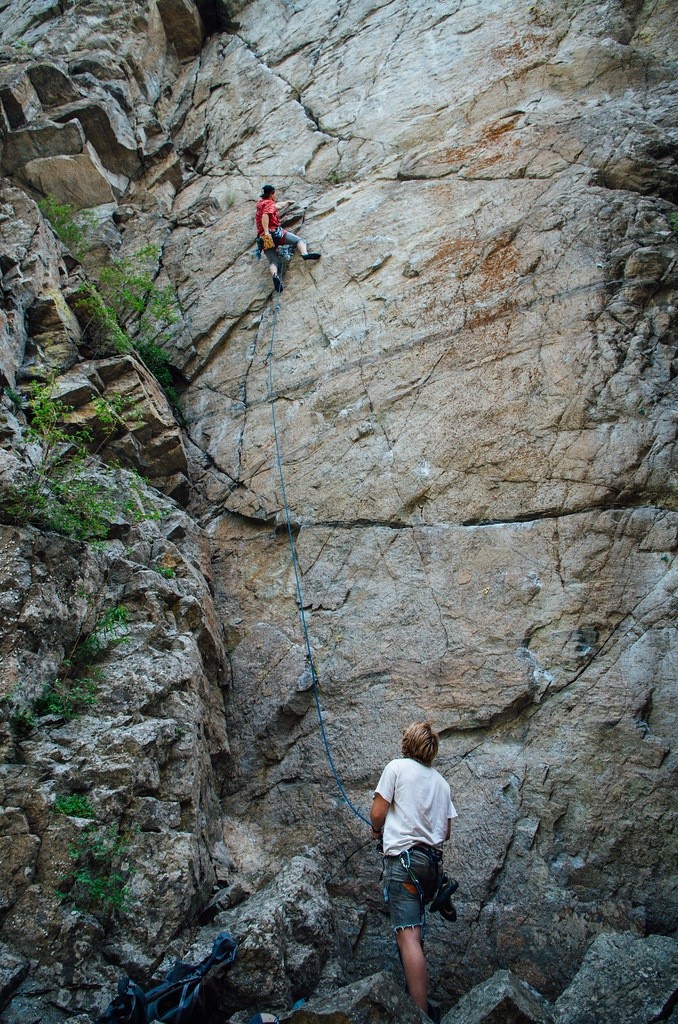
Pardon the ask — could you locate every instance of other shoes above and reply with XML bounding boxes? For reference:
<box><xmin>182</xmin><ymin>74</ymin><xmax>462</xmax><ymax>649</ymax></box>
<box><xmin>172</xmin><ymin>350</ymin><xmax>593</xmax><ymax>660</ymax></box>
<box><xmin>299</xmin><ymin>253</ymin><xmax>321</xmax><ymax>260</ymax></box>
<box><xmin>273</xmin><ymin>272</ymin><xmax>283</xmax><ymax>293</ymax></box>
<box><xmin>428</xmin><ymin>878</ymin><xmax>459</xmax><ymax>913</ymax></box>
<box><xmin>437</xmin><ymin>896</ymin><xmax>457</xmax><ymax>922</ymax></box>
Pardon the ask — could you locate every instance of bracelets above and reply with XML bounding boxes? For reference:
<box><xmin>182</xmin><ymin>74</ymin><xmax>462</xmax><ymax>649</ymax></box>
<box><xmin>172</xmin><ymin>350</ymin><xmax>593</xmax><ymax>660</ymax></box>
<box><xmin>372</xmin><ymin>825</ymin><xmax>381</xmax><ymax>833</ymax></box>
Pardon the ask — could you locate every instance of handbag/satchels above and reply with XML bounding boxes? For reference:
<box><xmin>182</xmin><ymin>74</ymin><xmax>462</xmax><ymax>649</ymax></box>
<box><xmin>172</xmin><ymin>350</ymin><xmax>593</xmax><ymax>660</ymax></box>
<box><xmin>263</xmin><ymin>234</ymin><xmax>275</xmax><ymax>249</ymax></box>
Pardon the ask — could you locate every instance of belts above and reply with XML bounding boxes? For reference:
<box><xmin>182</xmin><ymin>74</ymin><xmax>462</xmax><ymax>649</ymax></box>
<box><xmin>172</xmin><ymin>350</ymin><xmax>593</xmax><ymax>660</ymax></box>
<box><xmin>411</xmin><ymin>845</ymin><xmax>430</xmax><ymax>858</ymax></box>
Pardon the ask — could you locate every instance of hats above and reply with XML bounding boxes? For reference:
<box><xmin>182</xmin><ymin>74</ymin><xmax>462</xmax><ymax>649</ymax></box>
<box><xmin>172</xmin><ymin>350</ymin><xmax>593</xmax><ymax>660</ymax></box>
<box><xmin>259</xmin><ymin>185</ymin><xmax>275</xmax><ymax>197</ymax></box>
<box><xmin>248</xmin><ymin>1012</ymin><xmax>279</xmax><ymax>1024</ymax></box>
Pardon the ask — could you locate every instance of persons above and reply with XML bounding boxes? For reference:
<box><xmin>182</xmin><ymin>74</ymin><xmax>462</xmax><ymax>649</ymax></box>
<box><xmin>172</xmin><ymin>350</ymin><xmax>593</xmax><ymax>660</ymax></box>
<box><xmin>369</xmin><ymin>720</ymin><xmax>459</xmax><ymax>1018</ymax></box>
<box><xmin>255</xmin><ymin>185</ymin><xmax>321</xmax><ymax>292</ymax></box>
